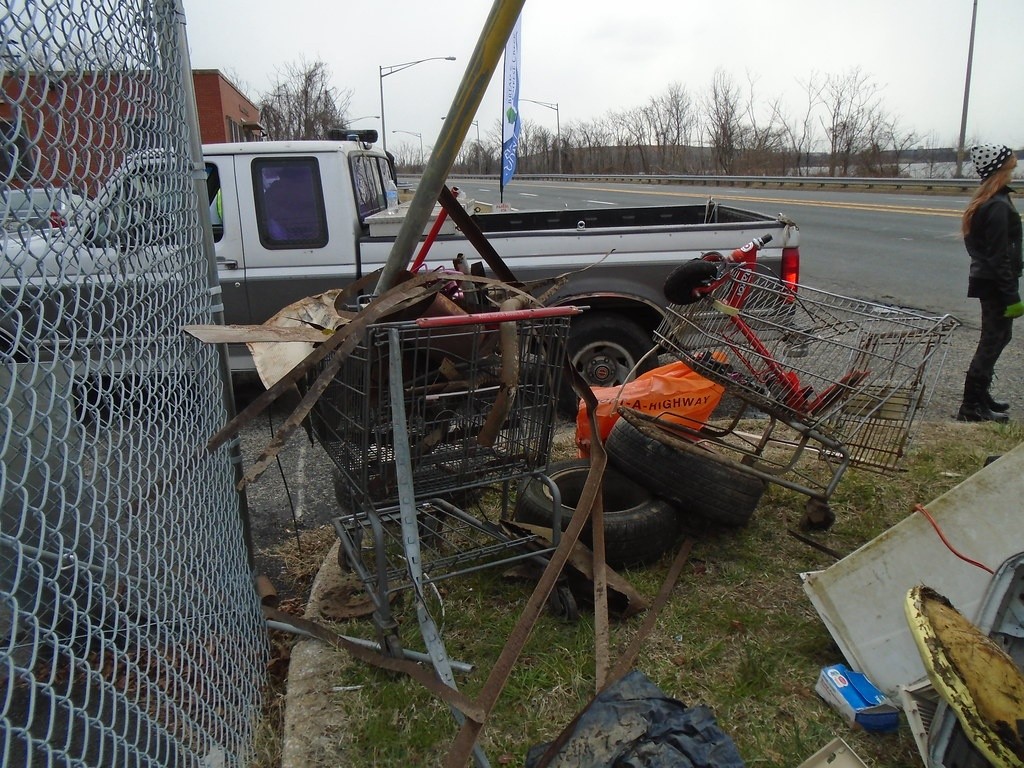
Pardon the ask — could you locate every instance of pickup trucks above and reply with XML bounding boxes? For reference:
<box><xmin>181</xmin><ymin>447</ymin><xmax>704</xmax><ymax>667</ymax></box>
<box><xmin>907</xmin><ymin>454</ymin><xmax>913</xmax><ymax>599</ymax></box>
<box><xmin>0</xmin><ymin>129</ymin><xmax>812</xmax><ymax>426</ymax></box>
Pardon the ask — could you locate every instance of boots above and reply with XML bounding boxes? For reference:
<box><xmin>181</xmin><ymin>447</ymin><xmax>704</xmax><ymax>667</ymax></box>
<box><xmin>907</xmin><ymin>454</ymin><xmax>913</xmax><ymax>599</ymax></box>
<box><xmin>988</xmin><ymin>372</ymin><xmax>1009</xmax><ymax>412</ymax></box>
<box><xmin>956</xmin><ymin>370</ymin><xmax>1008</xmax><ymax>422</ymax></box>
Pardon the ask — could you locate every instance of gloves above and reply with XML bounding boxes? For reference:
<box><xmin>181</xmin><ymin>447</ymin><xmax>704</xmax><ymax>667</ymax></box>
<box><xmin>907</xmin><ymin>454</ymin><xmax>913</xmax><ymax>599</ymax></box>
<box><xmin>1003</xmin><ymin>300</ymin><xmax>1024</xmax><ymax>318</ymax></box>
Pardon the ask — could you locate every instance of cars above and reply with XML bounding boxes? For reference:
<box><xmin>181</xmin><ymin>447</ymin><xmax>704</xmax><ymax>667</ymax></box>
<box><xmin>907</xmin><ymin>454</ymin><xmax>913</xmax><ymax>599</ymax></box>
<box><xmin>0</xmin><ymin>187</ymin><xmax>115</xmax><ymax>235</ymax></box>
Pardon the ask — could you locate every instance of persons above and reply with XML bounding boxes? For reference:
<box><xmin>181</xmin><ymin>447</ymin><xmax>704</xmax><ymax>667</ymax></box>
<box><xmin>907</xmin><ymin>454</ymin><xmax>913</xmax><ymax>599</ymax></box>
<box><xmin>210</xmin><ymin>187</ymin><xmax>222</xmax><ymax>225</ymax></box>
<box><xmin>956</xmin><ymin>142</ymin><xmax>1024</xmax><ymax>424</ymax></box>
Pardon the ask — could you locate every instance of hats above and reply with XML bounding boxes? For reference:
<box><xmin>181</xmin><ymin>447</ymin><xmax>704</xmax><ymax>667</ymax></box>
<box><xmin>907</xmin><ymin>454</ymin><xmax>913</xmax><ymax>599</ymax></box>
<box><xmin>971</xmin><ymin>143</ymin><xmax>1012</xmax><ymax>179</ymax></box>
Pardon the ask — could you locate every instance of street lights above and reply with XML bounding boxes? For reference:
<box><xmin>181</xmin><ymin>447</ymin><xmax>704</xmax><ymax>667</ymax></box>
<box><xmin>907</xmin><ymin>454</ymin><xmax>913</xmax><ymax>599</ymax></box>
<box><xmin>392</xmin><ymin>131</ymin><xmax>424</xmax><ymax>164</ymax></box>
<box><xmin>378</xmin><ymin>56</ymin><xmax>457</xmax><ymax>152</ymax></box>
<box><xmin>440</xmin><ymin>116</ymin><xmax>482</xmax><ymax>174</ymax></box>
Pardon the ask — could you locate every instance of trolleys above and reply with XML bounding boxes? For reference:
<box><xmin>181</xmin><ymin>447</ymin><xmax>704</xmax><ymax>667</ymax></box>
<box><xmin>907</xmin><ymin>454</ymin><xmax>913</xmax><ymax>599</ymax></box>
<box><xmin>289</xmin><ymin>305</ymin><xmax>585</xmax><ymax>680</ymax></box>
<box><xmin>614</xmin><ymin>257</ymin><xmax>958</xmax><ymax>541</ymax></box>
